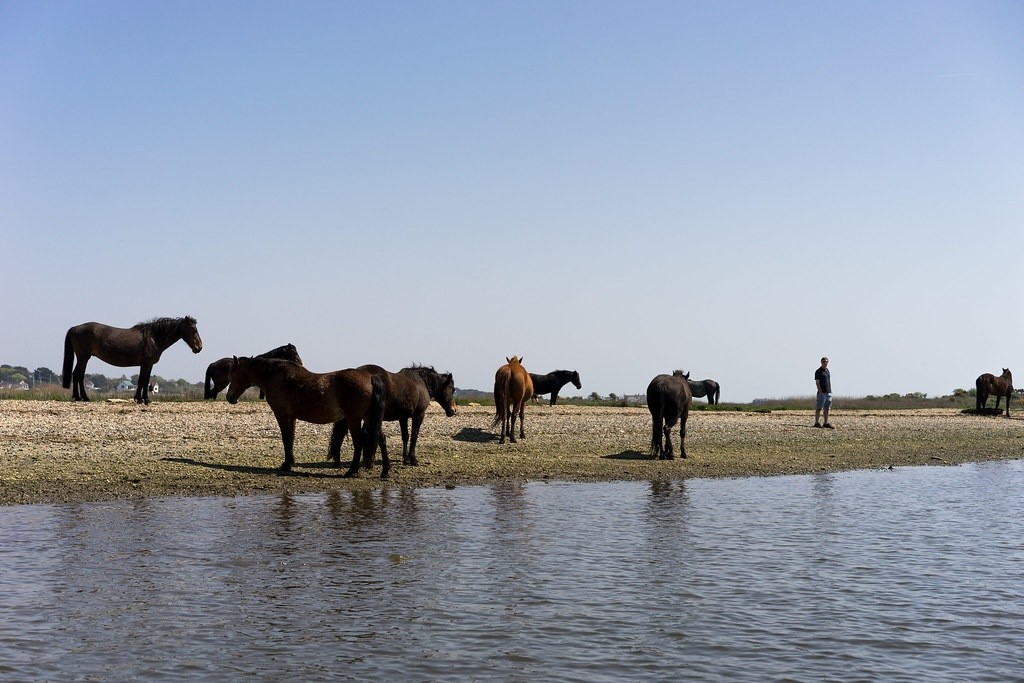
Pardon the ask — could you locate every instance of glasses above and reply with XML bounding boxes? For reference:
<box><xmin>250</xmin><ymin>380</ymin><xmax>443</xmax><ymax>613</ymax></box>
<box><xmin>821</xmin><ymin>361</ymin><xmax>828</xmax><ymax>363</ymax></box>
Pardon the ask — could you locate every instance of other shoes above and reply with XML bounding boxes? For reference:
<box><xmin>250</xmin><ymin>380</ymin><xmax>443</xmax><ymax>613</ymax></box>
<box><xmin>814</xmin><ymin>423</ymin><xmax>822</xmax><ymax>428</ymax></box>
<box><xmin>823</xmin><ymin>423</ymin><xmax>834</xmax><ymax>429</ymax></box>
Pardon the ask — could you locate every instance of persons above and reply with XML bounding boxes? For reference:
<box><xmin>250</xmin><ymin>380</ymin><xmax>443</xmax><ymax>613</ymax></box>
<box><xmin>815</xmin><ymin>357</ymin><xmax>835</xmax><ymax>429</ymax></box>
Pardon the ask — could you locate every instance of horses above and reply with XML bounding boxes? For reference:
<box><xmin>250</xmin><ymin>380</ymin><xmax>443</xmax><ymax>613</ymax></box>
<box><xmin>62</xmin><ymin>316</ymin><xmax>203</xmax><ymax>406</ymax></box>
<box><xmin>490</xmin><ymin>356</ymin><xmax>582</xmax><ymax>445</ymax></box>
<box><xmin>976</xmin><ymin>368</ymin><xmax>1013</xmax><ymax>417</ymax></box>
<box><xmin>647</xmin><ymin>370</ymin><xmax>720</xmax><ymax>460</ymax></box>
<box><xmin>203</xmin><ymin>344</ymin><xmax>457</xmax><ymax>478</ymax></box>
